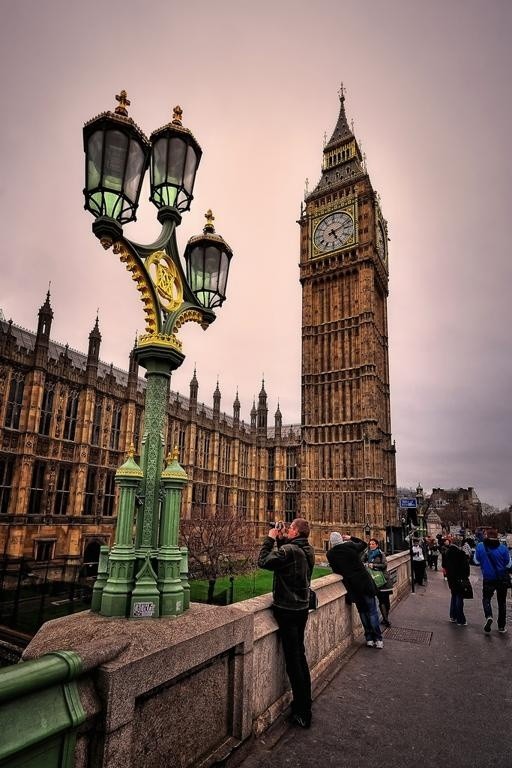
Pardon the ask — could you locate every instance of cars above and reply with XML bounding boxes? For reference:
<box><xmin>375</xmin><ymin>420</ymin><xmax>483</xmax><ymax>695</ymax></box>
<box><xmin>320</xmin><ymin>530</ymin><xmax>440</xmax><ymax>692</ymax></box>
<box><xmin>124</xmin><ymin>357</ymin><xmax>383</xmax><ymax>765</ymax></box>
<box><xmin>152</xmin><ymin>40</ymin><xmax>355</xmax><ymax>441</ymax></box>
<box><xmin>413</xmin><ymin>525</ymin><xmax>512</xmax><ymax>551</ymax></box>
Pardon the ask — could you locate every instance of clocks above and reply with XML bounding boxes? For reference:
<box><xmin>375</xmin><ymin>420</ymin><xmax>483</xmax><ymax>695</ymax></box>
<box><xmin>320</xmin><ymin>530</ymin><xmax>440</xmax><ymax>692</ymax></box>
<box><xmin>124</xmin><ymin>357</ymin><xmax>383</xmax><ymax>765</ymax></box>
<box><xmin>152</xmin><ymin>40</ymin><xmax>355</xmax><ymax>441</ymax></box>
<box><xmin>307</xmin><ymin>197</ymin><xmax>359</xmax><ymax>261</ymax></box>
<box><xmin>371</xmin><ymin>202</ymin><xmax>392</xmax><ymax>275</ymax></box>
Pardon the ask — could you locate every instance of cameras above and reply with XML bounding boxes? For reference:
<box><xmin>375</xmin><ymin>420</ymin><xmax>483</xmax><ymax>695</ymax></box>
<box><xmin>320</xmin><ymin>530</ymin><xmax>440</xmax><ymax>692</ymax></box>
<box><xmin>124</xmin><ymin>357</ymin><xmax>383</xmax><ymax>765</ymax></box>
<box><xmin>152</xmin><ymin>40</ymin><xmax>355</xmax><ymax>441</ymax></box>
<box><xmin>269</xmin><ymin>522</ymin><xmax>283</xmax><ymax>531</ymax></box>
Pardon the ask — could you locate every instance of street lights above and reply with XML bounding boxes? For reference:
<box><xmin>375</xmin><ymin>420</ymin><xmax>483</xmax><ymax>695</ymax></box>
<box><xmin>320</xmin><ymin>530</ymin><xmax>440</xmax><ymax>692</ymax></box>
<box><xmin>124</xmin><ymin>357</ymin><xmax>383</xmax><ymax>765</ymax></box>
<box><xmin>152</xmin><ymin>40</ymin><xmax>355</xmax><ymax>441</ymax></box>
<box><xmin>73</xmin><ymin>78</ymin><xmax>239</xmax><ymax>616</ymax></box>
<box><xmin>415</xmin><ymin>481</ymin><xmax>426</xmax><ymax>540</ymax></box>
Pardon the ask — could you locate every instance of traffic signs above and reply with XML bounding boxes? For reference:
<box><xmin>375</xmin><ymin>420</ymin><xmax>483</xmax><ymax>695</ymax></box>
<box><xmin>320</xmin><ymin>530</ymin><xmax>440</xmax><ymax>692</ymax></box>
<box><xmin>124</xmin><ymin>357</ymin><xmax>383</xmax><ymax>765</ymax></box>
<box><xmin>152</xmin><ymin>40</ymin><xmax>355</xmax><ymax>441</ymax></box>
<box><xmin>400</xmin><ymin>497</ymin><xmax>418</xmax><ymax>509</ymax></box>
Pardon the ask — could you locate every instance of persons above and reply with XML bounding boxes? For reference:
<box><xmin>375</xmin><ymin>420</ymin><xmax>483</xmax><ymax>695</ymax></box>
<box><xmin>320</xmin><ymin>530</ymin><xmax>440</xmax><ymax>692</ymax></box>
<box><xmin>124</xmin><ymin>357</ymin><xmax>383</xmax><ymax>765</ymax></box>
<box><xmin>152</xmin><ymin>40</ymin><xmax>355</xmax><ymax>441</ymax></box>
<box><xmin>410</xmin><ymin>527</ymin><xmax>512</xmax><ymax>634</ymax></box>
<box><xmin>361</xmin><ymin>539</ymin><xmax>393</xmax><ymax>627</ymax></box>
<box><xmin>325</xmin><ymin>531</ymin><xmax>385</xmax><ymax>649</ymax></box>
<box><xmin>257</xmin><ymin>518</ymin><xmax>315</xmax><ymax>729</ymax></box>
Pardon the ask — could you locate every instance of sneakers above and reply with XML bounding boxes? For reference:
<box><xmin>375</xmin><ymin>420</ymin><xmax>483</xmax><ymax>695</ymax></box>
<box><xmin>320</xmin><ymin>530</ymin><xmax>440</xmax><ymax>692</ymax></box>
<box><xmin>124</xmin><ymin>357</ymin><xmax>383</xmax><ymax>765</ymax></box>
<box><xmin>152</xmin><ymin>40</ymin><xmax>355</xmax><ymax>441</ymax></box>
<box><xmin>484</xmin><ymin>616</ymin><xmax>494</xmax><ymax>633</ymax></box>
<box><xmin>449</xmin><ymin>617</ymin><xmax>456</xmax><ymax>623</ymax></box>
<box><xmin>366</xmin><ymin>640</ymin><xmax>375</xmax><ymax>647</ymax></box>
<box><xmin>375</xmin><ymin>640</ymin><xmax>385</xmax><ymax>648</ymax></box>
<box><xmin>497</xmin><ymin>626</ymin><xmax>508</xmax><ymax>633</ymax></box>
<box><xmin>285</xmin><ymin>713</ymin><xmax>312</xmax><ymax>729</ymax></box>
<box><xmin>457</xmin><ymin>620</ymin><xmax>468</xmax><ymax>626</ymax></box>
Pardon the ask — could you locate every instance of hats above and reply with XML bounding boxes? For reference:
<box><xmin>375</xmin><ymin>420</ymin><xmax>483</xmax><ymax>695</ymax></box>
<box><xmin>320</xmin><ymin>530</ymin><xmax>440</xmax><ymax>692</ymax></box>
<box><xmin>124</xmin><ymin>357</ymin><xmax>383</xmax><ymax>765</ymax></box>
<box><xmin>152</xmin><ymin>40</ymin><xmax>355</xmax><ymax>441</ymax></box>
<box><xmin>451</xmin><ymin>536</ymin><xmax>462</xmax><ymax>546</ymax></box>
<box><xmin>329</xmin><ymin>531</ymin><xmax>343</xmax><ymax>545</ymax></box>
<box><xmin>486</xmin><ymin>529</ymin><xmax>498</xmax><ymax>538</ymax></box>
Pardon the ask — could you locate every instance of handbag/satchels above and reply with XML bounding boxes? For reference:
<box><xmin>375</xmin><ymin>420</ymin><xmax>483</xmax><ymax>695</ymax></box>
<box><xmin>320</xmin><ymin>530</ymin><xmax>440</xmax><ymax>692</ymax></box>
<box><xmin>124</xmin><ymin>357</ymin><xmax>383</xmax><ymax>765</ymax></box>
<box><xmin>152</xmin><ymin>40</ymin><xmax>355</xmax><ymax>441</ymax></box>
<box><xmin>366</xmin><ymin>567</ymin><xmax>388</xmax><ymax>588</ymax></box>
<box><xmin>308</xmin><ymin>587</ymin><xmax>318</xmax><ymax>612</ymax></box>
<box><xmin>461</xmin><ymin>576</ymin><xmax>474</xmax><ymax>600</ymax></box>
<box><xmin>495</xmin><ymin>567</ymin><xmax>512</xmax><ymax>589</ymax></box>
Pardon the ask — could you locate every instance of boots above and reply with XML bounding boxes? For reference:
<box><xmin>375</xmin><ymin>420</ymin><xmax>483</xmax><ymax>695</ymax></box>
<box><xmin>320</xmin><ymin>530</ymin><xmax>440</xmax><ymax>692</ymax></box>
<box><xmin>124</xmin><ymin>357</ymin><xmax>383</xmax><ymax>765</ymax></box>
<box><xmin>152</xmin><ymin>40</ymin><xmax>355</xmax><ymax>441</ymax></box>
<box><xmin>378</xmin><ymin>604</ymin><xmax>392</xmax><ymax>627</ymax></box>
